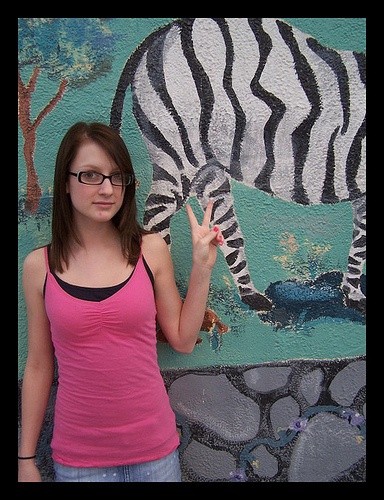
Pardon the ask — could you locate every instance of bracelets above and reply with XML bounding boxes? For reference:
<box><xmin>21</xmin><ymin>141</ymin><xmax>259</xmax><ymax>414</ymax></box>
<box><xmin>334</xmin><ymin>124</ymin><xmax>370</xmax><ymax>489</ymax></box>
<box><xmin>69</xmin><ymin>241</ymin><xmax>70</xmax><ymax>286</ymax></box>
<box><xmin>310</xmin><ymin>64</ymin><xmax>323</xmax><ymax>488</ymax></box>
<box><xmin>18</xmin><ymin>455</ymin><xmax>36</xmax><ymax>459</ymax></box>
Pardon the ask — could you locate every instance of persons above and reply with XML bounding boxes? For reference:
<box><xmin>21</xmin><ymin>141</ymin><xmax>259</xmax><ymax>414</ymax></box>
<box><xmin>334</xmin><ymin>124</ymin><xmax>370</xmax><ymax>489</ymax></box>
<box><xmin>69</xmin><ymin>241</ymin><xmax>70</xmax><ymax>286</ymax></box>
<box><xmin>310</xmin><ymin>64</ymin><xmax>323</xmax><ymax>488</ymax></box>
<box><xmin>17</xmin><ymin>121</ymin><xmax>224</xmax><ymax>482</ymax></box>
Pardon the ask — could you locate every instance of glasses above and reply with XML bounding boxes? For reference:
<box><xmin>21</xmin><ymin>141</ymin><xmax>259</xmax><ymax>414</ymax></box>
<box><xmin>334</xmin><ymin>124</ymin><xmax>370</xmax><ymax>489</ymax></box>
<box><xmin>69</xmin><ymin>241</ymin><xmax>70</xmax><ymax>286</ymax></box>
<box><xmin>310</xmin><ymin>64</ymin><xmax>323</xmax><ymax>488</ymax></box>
<box><xmin>69</xmin><ymin>170</ymin><xmax>134</xmax><ymax>186</ymax></box>
